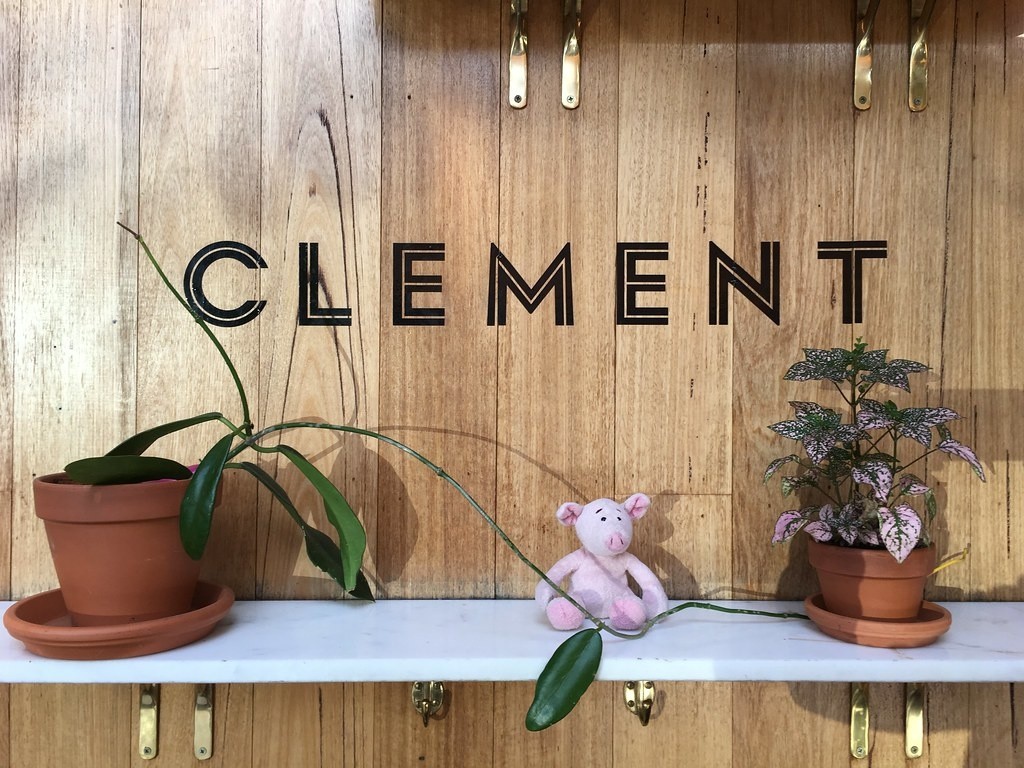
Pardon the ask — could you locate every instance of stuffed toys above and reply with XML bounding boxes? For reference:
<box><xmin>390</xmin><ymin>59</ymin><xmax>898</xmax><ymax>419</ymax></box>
<box><xmin>533</xmin><ymin>493</ymin><xmax>670</xmax><ymax>631</ymax></box>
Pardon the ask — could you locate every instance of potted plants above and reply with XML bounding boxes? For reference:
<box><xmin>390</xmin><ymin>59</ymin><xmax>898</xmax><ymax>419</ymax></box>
<box><xmin>759</xmin><ymin>337</ymin><xmax>987</xmax><ymax>623</ymax></box>
<box><xmin>35</xmin><ymin>223</ymin><xmax>806</xmax><ymax>731</ymax></box>
